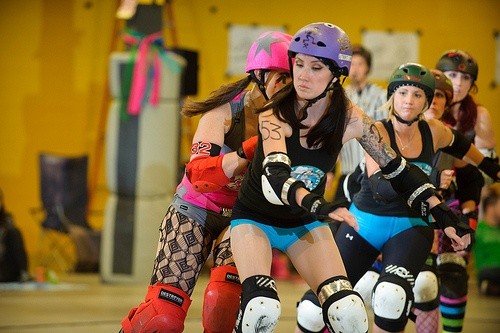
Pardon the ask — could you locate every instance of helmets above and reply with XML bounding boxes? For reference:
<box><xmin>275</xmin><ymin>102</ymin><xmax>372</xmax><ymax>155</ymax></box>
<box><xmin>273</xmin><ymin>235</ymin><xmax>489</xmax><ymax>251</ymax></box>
<box><xmin>386</xmin><ymin>62</ymin><xmax>436</xmax><ymax>97</ymax></box>
<box><xmin>436</xmin><ymin>49</ymin><xmax>478</xmax><ymax>81</ymax></box>
<box><xmin>244</xmin><ymin>30</ymin><xmax>295</xmax><ymax>74</ymax></box>
<box><xmin>430</xmin><ymin>68</ymin><xmax>455</xmax><ymax>106</ymax></box>
<box><xmin>286</xmin><ymin>22</ymin><xmax>353</xmax><ymax>77</ymax></box>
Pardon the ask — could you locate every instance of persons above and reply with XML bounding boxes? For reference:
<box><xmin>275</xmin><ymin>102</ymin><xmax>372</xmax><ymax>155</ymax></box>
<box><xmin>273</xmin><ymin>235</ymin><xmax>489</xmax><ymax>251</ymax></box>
<box><xmin>118</xmin><ymin>22</ymin><xmax>500</xmax><ymax>333</ymax></box>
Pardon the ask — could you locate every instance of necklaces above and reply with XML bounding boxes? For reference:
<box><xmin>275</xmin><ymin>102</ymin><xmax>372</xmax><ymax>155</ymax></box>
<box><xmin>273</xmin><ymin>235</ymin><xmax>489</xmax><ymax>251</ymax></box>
<box><xmin>395</xmin><ymin>130</ymin><xmax>418</xmax><ymax>150</ymax></box>
